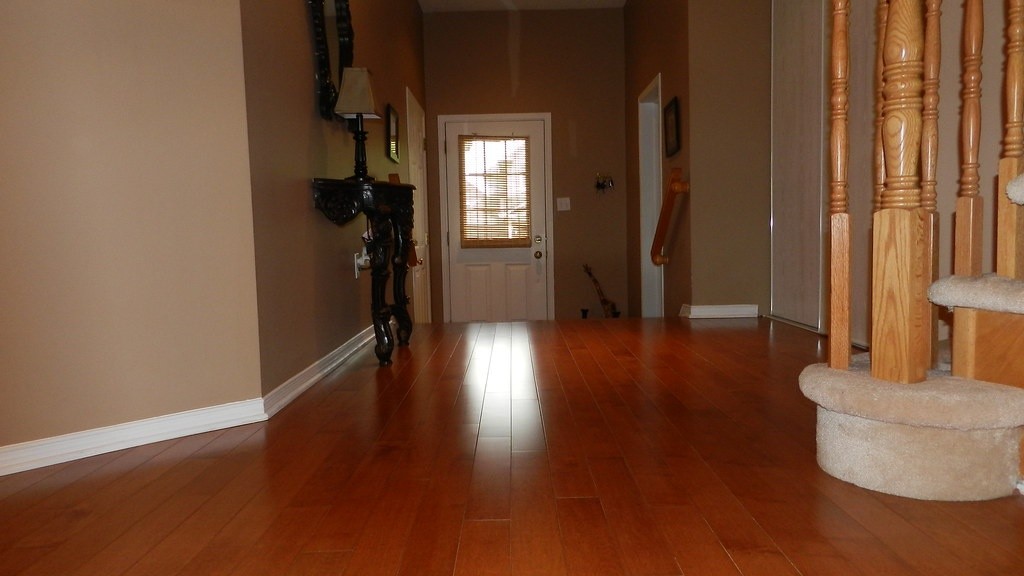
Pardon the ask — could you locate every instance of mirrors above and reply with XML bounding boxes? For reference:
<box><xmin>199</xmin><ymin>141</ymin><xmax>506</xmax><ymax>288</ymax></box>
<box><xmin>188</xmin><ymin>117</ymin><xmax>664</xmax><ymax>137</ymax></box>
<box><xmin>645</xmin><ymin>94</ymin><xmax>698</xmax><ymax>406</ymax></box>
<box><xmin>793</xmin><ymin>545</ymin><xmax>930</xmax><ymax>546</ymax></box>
<box><xmin>308</xmin><ymin>0</ymin><xmax>355</xmax><ymax>121</ymax></box>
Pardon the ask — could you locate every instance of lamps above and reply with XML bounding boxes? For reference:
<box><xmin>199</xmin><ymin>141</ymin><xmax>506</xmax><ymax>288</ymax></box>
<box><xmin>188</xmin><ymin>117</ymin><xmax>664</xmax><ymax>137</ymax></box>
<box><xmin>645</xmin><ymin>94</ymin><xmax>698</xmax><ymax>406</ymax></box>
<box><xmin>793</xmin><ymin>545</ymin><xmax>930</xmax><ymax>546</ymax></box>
<box><xmin>592</xmin><ymin>169</ymin><xmax>616</xmax><ymax>194</ymax></box>
<box><xmin>333</xmin><ymin>67</ymin><xmax>382</xmax><ymax>182</ymax></box>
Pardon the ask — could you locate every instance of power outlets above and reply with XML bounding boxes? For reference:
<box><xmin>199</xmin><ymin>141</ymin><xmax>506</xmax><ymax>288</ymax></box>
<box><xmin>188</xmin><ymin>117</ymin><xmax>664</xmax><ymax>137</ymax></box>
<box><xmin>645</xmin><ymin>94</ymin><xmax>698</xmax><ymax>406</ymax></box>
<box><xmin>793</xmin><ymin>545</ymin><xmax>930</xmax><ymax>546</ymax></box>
<box><xmin>354</xmin><ymin>252</ymin><xmax>361</xmax><ymax>279</ymax></box>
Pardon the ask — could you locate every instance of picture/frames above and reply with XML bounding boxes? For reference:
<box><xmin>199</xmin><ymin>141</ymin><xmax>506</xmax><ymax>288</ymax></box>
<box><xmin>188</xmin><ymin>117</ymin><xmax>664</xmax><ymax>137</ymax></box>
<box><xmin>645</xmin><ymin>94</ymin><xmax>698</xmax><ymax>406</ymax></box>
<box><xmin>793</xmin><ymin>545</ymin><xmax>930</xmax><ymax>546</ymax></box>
<box><xmin>663</xmin><ymin>97</ymin><xmax>681</xmax><ymax>157</ymax></box>
<box><xmin>386</xmin><ymin>103</ymin><xmax>401</xmax><ymax>164</ymax></box>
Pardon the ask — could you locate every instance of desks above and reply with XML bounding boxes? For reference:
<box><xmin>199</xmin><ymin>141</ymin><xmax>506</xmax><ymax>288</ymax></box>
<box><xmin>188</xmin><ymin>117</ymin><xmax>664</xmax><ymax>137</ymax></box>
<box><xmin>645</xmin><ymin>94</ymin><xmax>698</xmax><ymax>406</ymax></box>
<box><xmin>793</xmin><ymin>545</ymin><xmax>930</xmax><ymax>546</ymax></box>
<box><xmin>313</xmin><ymin>177</ymin><xmax>417</xmax><ymax>366</ymax></box>
